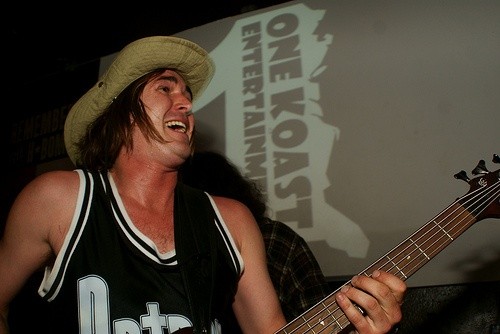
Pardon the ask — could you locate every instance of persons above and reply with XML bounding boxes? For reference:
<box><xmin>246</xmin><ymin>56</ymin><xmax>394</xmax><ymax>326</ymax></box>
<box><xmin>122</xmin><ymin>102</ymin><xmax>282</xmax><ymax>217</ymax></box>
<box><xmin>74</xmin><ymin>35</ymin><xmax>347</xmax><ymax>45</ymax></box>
<box><xmin>176</xmin><ymin>151</ymin><xmax>331</xmax><ymax>324</ymax></box>
<box><xmin>0</xmin><ymin>35</ymin><xmax>408</xmax><ymax>334</ymax></box>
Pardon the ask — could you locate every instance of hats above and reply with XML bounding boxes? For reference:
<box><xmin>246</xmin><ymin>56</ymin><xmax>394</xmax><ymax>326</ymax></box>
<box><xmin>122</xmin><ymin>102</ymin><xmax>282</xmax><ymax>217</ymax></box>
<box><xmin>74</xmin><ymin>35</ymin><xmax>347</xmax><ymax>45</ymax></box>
<box><xmin>63</xmin><ymin>33</ymin><xmax>217</xmax><ymax>172</ymax></box>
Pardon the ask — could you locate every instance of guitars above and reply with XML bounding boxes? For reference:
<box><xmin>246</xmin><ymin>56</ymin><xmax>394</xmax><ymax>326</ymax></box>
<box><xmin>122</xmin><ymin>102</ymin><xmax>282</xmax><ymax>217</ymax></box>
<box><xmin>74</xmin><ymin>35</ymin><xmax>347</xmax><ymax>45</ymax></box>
<box><xmin>270</xmin><ymin>152</ymin><xmax>500</xmax><ymax>334</ymax></box>
